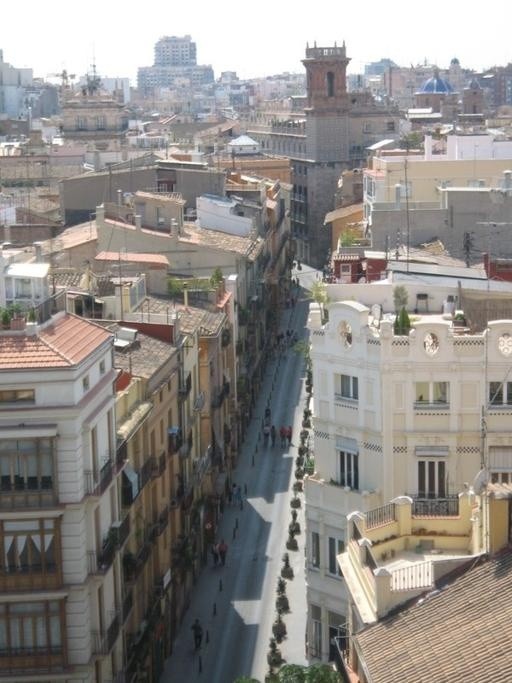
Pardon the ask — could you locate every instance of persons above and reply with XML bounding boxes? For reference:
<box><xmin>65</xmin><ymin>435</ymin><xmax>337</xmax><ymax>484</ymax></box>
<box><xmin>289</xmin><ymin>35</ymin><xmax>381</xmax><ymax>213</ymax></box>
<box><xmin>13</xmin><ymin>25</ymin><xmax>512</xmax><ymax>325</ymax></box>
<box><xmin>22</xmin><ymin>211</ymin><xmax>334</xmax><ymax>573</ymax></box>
<box><xmin>189</xmin><ymin>618</ymin><xmax>203</xmax><ymax>648</ymax></box>
<box><xmin>232</xmin><ymin>482</ymin><xmax>237</xmax><ymax>500</ymax></box>
<box><xmin>211</xmin><ymin>541</ymin><xmax>219</xmax><ymax>567</ymax></box>
<box><xmin>219</xmin><ymin>538</ymin><xmax>228</xmax><ymax>564</ymax></box>
<box><xmin>263</xmin><ymin>405</ymin><xmax>291</xmax><ymax>449</ymax></box>
